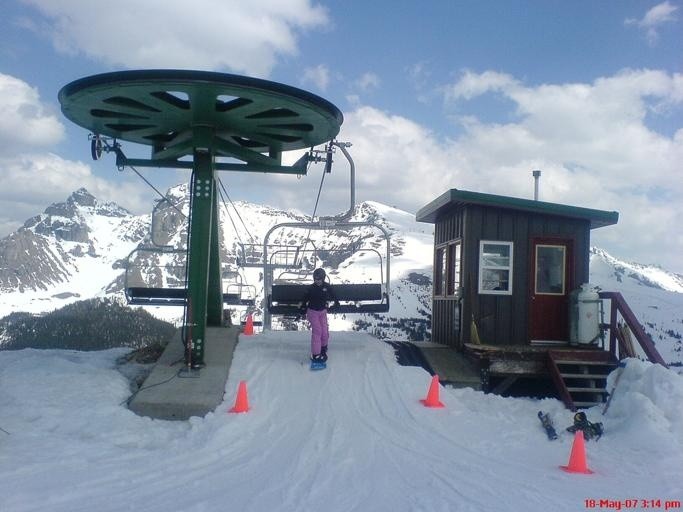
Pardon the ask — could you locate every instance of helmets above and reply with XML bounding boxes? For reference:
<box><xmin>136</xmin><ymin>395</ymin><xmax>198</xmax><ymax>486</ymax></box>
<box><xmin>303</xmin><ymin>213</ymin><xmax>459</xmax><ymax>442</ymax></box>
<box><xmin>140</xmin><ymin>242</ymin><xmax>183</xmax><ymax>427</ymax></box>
<box><xmin>313</xmin><ymin>268</ymin><xmax>326</xmax><ymax>279</ymax></box>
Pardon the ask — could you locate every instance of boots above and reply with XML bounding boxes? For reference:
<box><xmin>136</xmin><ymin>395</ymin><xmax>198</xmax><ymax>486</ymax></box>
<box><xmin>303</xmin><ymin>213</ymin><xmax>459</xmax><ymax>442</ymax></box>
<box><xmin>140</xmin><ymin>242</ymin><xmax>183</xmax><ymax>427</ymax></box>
<box><xmin>311</xmin><ymin>345</ymin><xmax>328</xmax><ymax>363</ymax></box>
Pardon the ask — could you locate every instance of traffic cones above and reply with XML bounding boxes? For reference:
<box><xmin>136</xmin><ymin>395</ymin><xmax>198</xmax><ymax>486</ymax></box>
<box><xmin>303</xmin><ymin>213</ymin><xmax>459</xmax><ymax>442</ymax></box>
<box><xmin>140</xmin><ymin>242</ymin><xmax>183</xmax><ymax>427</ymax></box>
<box><xmin>228</xmin><ymin>380</ymin><xmax>252</xmax><ymax>413</ymax></box>
<box><xmin>243</xmin><ymin>313</ymin><xmax>254</xmax><ymax>335</ymax></box>
<box><xmin>419</xmin><ymin>374</ymin><xmax>445</xmax><ymax>408</ymax></box>
<box><xmin>559</xmin><ymin>430</ymin><xmax>593</xmax><ymax>474</ymax></box>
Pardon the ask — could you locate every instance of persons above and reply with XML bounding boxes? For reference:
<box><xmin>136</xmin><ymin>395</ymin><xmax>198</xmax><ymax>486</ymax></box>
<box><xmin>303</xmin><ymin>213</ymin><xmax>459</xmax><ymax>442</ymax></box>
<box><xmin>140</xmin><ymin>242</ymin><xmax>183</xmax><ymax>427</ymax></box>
<box><xmin>300</xmin><ymin>268</ymin><xmax>340</xmax><ymax>361</ymax></box>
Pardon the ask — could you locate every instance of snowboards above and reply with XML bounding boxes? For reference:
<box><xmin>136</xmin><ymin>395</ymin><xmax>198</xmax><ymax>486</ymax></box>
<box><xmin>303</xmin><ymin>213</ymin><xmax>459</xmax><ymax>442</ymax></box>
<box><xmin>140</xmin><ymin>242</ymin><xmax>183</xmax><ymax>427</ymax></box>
<box><xmin>312</xmin><ymin>359</ymin><xmax>325</xmax><ymax>370</ymax></box>
